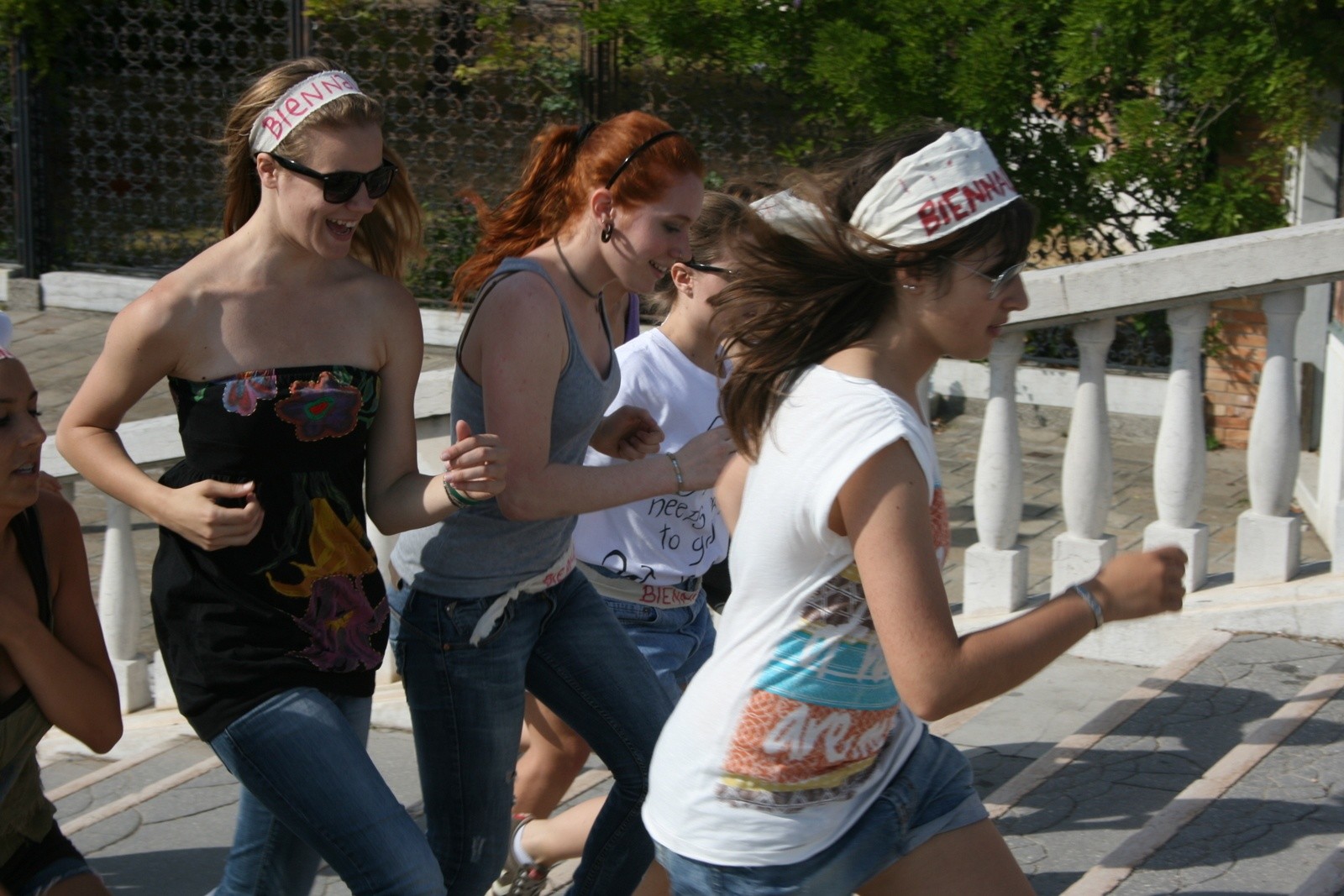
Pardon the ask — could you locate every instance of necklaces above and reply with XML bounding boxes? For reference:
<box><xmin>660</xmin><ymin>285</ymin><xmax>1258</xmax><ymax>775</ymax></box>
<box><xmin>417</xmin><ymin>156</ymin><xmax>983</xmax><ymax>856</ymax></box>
<box><xmin>554</xmin><ymin>234</ymin><xmax>602</xmax><ymax>298</ymax></box>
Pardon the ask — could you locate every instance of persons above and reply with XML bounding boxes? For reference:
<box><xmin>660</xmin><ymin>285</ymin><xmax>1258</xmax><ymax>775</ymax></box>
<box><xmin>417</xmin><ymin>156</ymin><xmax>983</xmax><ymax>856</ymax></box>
<box><xmin>641</xmin><ymin>114</ymin><xmax>1190</xmax><ymax>896</ymax></box>
<box><xmin>517</xmin><ymin>275</ymin><xmax>640</xmax><ymax>758</ymax></box>
<box><xmin>503</xmin><ymin>188</ymin><xmax>775</xmax><ymax>896</ymax></box>
<box><xmin>0</xmin><ymin>316</ymin><xmax>124</xmax><ymax>896</ymax></box>
<box><xmin>395</xmin><ymin>110</ymin><xmax>749</xmax><ymax>896</ymax></box>
<box><xmin>53</xmin><ymin>54</ymin><xmax>508</xmax><ymax>896</ymax></box>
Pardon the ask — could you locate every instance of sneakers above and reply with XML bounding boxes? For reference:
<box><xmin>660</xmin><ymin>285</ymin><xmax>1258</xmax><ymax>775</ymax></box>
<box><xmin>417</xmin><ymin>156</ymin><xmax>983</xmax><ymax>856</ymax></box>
<box><xmin>483</xmin><ymin>813</ymin><xmax>561</xmax><ymax>896</ymax></box>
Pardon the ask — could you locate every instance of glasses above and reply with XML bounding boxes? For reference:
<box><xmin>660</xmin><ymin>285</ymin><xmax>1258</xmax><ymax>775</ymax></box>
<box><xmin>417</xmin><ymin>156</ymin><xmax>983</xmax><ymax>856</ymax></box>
<box><xmin>255</xmin><ymin>151</ymin><xmax>399</xmax><ymax>204</ymax></box>
<box><xmin>689</xmin><ymin>264</ymin><xmax>744</xmax><ymax>282</ymax></box>
<box><xmin>930</xmin><ymin>251</ymin><xmax>1031</xmax><ymax>300</ymax></box>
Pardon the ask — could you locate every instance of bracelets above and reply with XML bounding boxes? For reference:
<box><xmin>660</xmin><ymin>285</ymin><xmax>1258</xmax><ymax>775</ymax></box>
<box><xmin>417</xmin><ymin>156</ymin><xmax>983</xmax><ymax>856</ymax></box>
<box><xmin>445</xmin><ymin>470</ymin><xmax>477</xmax><ymax>505</ymax></box>
<box><xmin>1065</xmin><ymin>585</ymin><xmax>1103</xmax><ymax>629</ymax></box>
<box><xmin>667</xmin><ymin>452</ymin><xmax>685</xmax><ymax>493</ymax></box>
<box><xmin>443</xmin><ymin>482</ymin><xmax>463</xmax><ymax>508</ymax></box>
